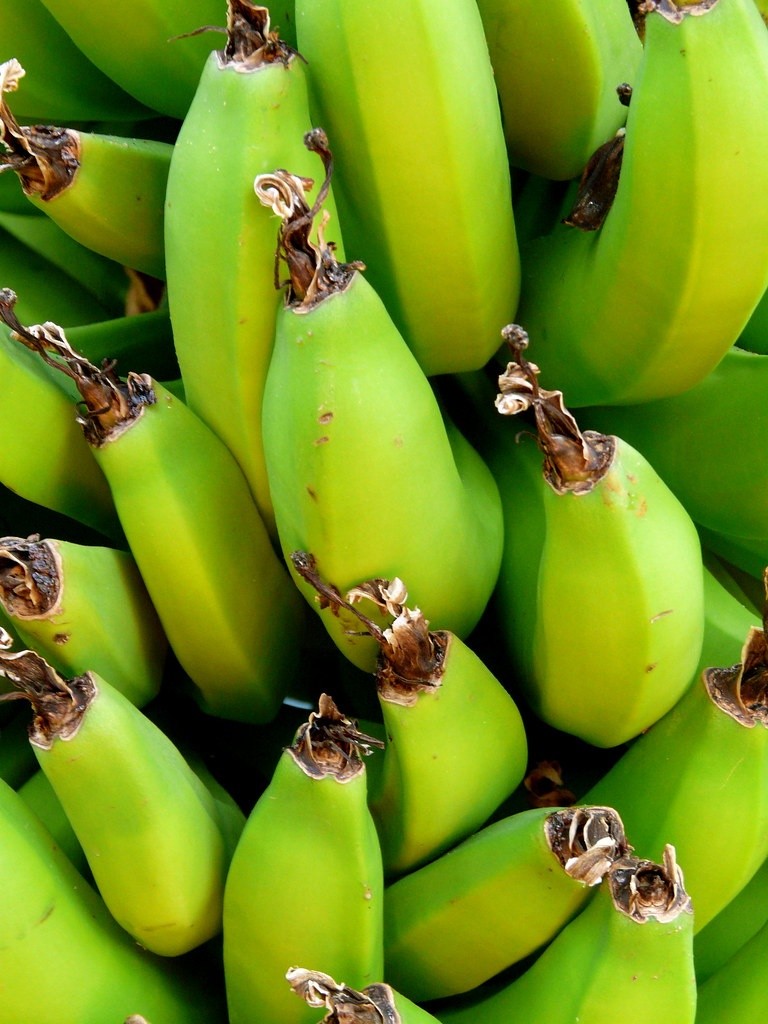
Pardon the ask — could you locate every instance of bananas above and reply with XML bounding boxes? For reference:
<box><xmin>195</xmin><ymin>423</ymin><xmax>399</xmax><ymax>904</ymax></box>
<box><xmin>1</xmin><ymin>0</ymin><xmax>768</xmax><ymax>1024</ymax></box>
